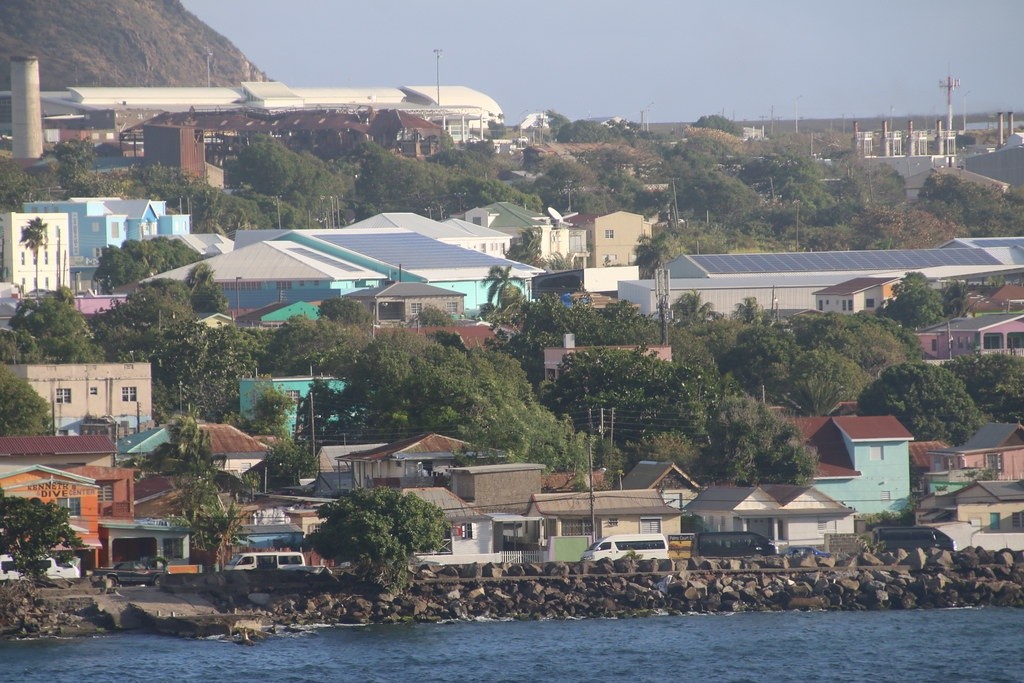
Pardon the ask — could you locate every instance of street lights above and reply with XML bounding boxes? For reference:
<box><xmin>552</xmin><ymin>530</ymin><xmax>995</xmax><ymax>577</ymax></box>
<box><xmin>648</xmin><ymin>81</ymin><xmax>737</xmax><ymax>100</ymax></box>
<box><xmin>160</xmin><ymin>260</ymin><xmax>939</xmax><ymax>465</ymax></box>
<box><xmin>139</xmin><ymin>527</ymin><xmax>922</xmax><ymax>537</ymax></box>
<box><xmin>234</xmin><ymin>277</ymin><xmax>244</xmax><ymax>318</ymax></box>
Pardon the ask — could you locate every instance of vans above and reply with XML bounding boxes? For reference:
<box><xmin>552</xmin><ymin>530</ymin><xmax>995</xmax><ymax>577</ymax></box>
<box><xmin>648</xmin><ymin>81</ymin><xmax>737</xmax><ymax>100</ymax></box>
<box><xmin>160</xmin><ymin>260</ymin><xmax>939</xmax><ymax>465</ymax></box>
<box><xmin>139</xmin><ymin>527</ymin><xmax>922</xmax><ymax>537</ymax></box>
<box><xmin>224</xmin><ymin>551</ymin><xmax>307</xmax><ymax>571</ymax></box>
<box><xmin>667</xmin><ymin>532</ymin><xmax>695</xmax><ymax>560</ymax></box>
<box><xmin>690</xmin><ymin>531</ymin><xmax>777</xmax><ymax>557</ymax></box>
<box><xmin>580</xmin><ymin>532</ymin><xmax>671</xmax><ymax>562</ymax></box>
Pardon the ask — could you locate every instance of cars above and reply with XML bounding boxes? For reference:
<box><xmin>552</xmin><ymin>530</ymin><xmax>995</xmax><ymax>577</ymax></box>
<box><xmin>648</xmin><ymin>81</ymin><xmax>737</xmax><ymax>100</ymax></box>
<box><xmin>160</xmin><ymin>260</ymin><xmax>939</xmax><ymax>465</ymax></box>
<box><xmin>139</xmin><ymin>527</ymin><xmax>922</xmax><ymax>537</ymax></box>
<box><xmin>92</xmin><ymin>561</ymin><xmax>165</xmax><ymax>589</ymax></box>
<box><xmin>298</xmin><ymin>565</ymin><xmax>334</xmax><ymax>576</ymax></box>
<box><xmin>780</xmin><ymin>546</ymin><xmax>832</xmax><ymax>558</ymax></box>
